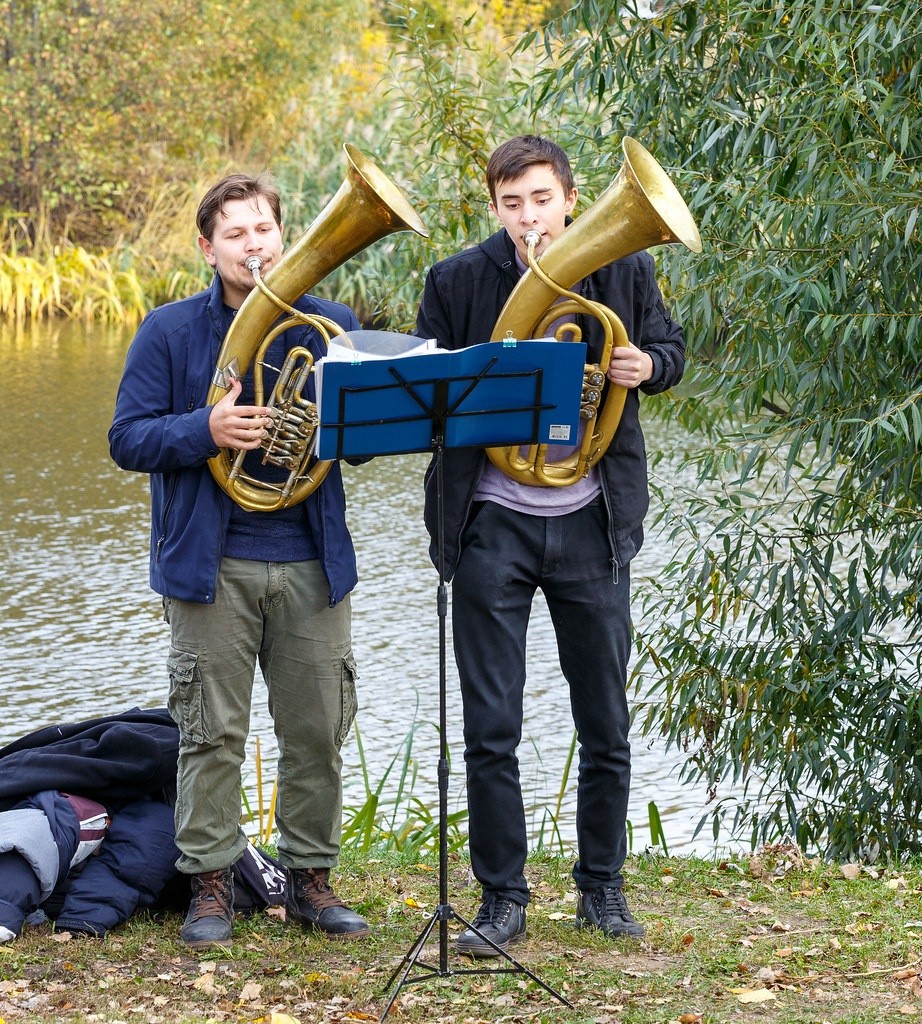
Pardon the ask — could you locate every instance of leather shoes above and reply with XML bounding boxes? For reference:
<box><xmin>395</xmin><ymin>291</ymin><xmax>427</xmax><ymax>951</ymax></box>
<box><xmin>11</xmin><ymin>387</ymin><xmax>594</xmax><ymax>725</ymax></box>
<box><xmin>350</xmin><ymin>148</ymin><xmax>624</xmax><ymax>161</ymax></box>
<box><xmin>286</xmin><ymin>868</ymin><xmax>370</xmax><ymax>941</ymax></box>
<box><xmin>456</xmin><ymin>895</ymin><xmax>526</xmax><ymax>955</ymax></box>
<box><xmin>575</xmin><ymin>885</ymin><xmax>646</xmax><ymax>940</ymax></box>
<box><xmin>181</xmin><ymin>869</ymin><xmax>233</xmax><ymax>951</ymax></box>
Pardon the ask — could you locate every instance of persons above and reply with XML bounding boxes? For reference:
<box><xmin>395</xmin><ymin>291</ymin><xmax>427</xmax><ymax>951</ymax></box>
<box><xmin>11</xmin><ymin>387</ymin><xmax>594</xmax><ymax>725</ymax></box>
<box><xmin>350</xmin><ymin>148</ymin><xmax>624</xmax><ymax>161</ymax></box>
<box><xmin>410</xmin><ymin>135</ymin><xmax>687</xmax><ymax>955</ymax></box>
<box><xmin>109</xmin><ymin>173</ymin><xmax>373</xmax><ymax>950</ymax></box>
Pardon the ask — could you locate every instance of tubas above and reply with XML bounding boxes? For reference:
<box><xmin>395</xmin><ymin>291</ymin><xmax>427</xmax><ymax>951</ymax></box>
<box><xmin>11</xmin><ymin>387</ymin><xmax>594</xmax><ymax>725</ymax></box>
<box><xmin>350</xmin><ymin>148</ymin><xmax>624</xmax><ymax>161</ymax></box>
<box><xmin>479</xmin><ymin>135</ymin><xmax>708</xmax><ymax>489</ymax></box>
<box><xmin>201</xmin><ymin>145</ymin><xmax>433</xmax><ymax>512</ymax></box>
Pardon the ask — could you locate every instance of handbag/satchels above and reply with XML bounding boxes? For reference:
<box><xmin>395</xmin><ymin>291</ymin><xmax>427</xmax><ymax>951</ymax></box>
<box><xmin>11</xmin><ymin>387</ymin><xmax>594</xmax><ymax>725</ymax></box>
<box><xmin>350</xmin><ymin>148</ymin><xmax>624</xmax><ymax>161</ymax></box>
<box><xmin>230</xmin><ymin>840</ymin><xmax>288</xmax><ymax>918</ymax></box>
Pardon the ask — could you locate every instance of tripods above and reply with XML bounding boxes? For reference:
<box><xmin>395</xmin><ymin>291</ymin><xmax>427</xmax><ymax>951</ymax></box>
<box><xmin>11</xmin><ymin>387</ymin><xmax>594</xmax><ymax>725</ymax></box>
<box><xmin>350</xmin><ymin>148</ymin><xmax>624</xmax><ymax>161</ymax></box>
<box><xmin>315</xmin><ymin>338</ymin><xmax>587</xmax><ymax>1024</ymax></box>
